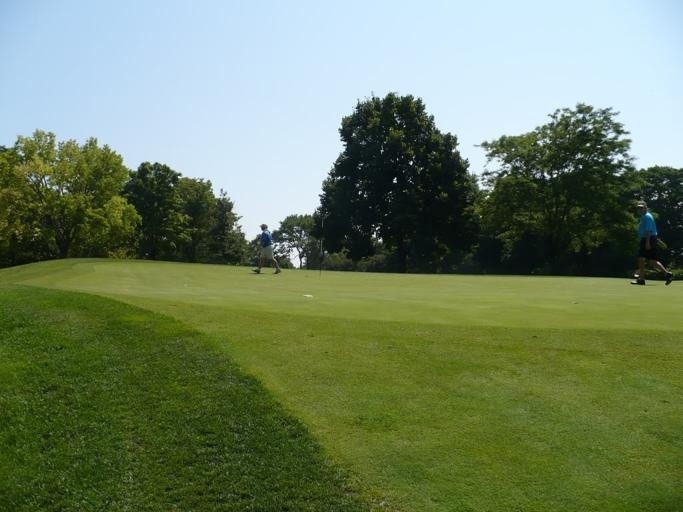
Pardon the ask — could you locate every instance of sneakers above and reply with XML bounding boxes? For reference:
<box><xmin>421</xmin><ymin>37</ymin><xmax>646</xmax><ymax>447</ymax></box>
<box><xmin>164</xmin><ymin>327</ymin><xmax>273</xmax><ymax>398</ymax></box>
<box><xmin>253</xmin><ymin>268</ymin><xmax>260</xmax><ymax>273</ymax></box>
<box><xmin>665</xmin><ymin>273</ymin><xmax>674</xmax><ymax>284</ymax></box>
<box><xmin>632</xmin><ymin>279</ymin><xmax>646</xmax><ymax>285</ymax></box>
<box><xmin>274</xmin><ymin>269</ymin><xmax>281</xmax><ymax>274</ymax></box>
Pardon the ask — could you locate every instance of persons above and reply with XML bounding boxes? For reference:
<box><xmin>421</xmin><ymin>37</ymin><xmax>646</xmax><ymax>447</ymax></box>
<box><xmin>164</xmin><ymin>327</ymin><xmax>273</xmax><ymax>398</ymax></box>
<box><xmin>251</xmin><ymin>223</ymin><xmax>282</xmax><ymax>275</ymax></box>
<box><xmin>630</xmin><ymin>200</ymin><xmax>673</xmax><ymax>285</ymax></box>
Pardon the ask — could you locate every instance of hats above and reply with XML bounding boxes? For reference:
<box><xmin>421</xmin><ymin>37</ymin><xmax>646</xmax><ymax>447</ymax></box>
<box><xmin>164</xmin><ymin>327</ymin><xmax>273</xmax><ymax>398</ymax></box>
<box><xmin>636</xmin><ymin>201</ymin><xmax>647</xmax><ymax>207</ymax></box>
<box><xmin>260</xmin><ymin>224</ymin><xmax>267</xmax><ymax>228</ymax></box>
